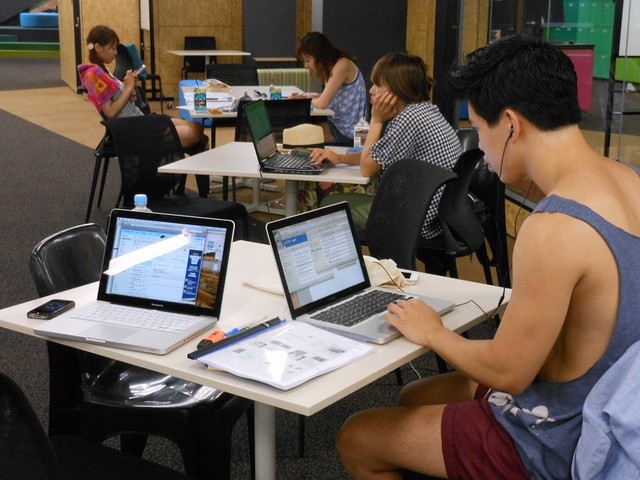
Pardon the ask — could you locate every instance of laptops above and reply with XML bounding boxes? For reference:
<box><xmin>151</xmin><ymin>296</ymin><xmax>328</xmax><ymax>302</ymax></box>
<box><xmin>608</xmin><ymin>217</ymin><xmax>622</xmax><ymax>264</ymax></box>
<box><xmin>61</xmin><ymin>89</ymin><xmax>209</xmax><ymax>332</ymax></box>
<box><xmin>32</xmin><ymin>206</ymin><xmax>237</xmax><ymax>356</ymax></box>
<box><xmin>241</xmin><ymin>97</ymin><xmax>328</xmax><ymax>176</ymax></box>
<box><xmin>265</xmin><ymin>201</ymin><xmax>458</xmax><ymax>346</ymax></box>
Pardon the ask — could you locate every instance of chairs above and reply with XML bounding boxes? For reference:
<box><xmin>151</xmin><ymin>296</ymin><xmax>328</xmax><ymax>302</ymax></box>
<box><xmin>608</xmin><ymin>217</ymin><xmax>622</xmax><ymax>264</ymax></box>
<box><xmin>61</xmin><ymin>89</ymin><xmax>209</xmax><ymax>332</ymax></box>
<box><xmin>0</xmin><ymin>371</ymin><xmax>191</xmax><ymax>480</ymax></box>
<box><xmin>357</xmin><ymin>149</ymin><xmax>486</xmax><ymax>285</ymax></box>
<box><xmin>447</xmin><ymin>128</ymin><xmax>511</xmax><ymax>288</ymax></box>
<box><xmin>28</xmin><ymin>222</ymin><xmax>255</xmax><ymax>480</ymax></box>
<box><xmin>182</xmin><ymin>36</ymin><xmax>218</xmax><ymax>80</ymax></box>
<box><xmin>100</xmin><ymin>113</ymin><xmax>249</xmax><ymax>240</ymax></box>
<box><xmin>77</xmin><ymin>85</ymin><xmax>210</xmax><ymax>223</ymax></box>
<box><xmin>296</xmin><ymin>160</ymin><xmax>458</xmax><ymax>456</ymax></box>
<box><xmin>113</xmin><ymin>44</ymin><xmax>163</xmax><ymax>102</ymax></box>
<box><xmin>223</xmin><ymin>99</ymin><xmax>312</xmax><ymax>200</ymax></box>
<box><xmin>200</xmin><ymin>62</ymin><xmax>259</xmax><ymax>148</ymax></box>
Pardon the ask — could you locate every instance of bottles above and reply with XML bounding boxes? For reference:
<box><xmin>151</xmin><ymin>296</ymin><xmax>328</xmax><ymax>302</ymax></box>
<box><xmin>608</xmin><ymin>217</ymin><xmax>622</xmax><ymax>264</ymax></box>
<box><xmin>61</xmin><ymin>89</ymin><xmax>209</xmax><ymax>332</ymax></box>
<box><xmin>353</xmin><ymin>116</ymin><xmax>369</xmax><ymax>148</ymax></box>
<box><xmin>193</xmin><ymin>87</ymin><xmax>206</xmax><ymax>113</ymax></box>
<box><xmin>269</xmin><ymin>87</ymin><xmax>282</xmax><ymax>100</ymax></box>
<box><xmin>131</xmin><ymin>194</ymin><xmax>152</xmax><ymax>213</ymax></box>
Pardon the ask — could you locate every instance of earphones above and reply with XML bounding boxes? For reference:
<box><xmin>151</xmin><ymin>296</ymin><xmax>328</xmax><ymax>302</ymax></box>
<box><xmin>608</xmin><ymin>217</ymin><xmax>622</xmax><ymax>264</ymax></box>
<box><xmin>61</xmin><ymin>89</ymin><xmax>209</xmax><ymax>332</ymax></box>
<box><xmin>505</xmin><ymin>124</ymin><xmax>519</xmax><ymax>143</ymax></box>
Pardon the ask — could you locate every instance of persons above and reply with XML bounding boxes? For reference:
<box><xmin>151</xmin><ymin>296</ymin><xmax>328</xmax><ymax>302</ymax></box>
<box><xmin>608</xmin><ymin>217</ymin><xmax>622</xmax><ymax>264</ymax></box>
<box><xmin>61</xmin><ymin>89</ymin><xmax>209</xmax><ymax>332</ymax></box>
<box><xmin>334</xmin><ymin>34</ymin><xmax>640</xmax><ymax>479</ymax></box>
<box><xmin>78</xmin><ymin>26</ymin><xmax>212</xmax><ymax>199</ymax></box>
<box><xmin>288</xmin><ymin>31</ymin><xmax>369</xmax><ymax>144</ymax></box>
<box><xmin>308</xmin><ymin>52</ymin><xmax>465</xmax><ymax>242</ymax></box>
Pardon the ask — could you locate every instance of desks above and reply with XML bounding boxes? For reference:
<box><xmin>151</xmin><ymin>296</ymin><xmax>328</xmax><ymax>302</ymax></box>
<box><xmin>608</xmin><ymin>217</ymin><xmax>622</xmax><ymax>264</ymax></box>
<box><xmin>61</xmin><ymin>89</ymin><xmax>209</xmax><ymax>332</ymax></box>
<box><xmin>165</xmin><ymin>48</ymin><xmax>251</xmax><ymax>80</ymax></box>
<box><xmin>0</xmin><ymin>239</ymin><xmax>512</xmax><ymax>480</ymax></box>
<box><xmin>181</xmin><ymin>85</ymin><xmax>335</xmax><ymax>194</ymax></box>
<box><xmin>156</xmin><ymin>141</ymin><xmax>379</xmax><ymax>217</ymax></box>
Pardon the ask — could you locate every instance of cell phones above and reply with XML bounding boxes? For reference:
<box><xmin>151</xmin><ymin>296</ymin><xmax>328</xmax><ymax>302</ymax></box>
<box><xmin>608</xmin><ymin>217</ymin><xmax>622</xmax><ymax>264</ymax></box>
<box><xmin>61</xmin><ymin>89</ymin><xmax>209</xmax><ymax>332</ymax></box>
<box><xmin>133</xmin><ymin>62</ymin><xmax>148</xmax><ymax>78</ymax></box>
<box><xmin>399</xmin><ymin>268</ymin><xmax>421</xmax><ymax>283</ymax></box>
<box><xmin>27</xmin><ymin>298</ymin><xmax>75</xmax><ymax>319</ymax></box>
